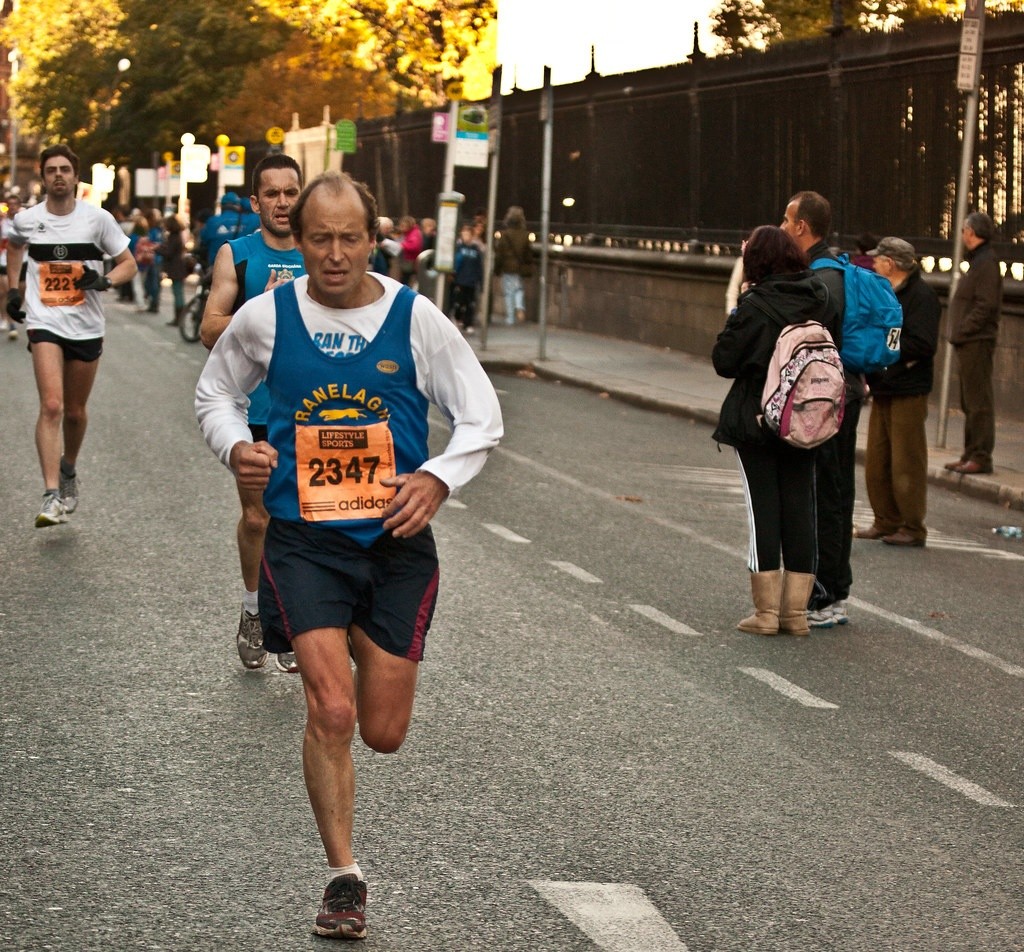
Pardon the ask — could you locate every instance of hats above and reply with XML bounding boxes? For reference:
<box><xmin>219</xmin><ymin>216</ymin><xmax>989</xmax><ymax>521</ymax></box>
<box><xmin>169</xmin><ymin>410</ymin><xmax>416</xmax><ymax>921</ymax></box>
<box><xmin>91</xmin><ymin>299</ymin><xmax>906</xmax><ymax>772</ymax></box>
<box><xmin>865</xmin><ymin>236</ymin><xmax>915</xmax><ymax>264</ymax></box>
<box><xmin>221</xmin><ymin>191</ymin><xmax>241</xmax><ymax>205</ymax></box>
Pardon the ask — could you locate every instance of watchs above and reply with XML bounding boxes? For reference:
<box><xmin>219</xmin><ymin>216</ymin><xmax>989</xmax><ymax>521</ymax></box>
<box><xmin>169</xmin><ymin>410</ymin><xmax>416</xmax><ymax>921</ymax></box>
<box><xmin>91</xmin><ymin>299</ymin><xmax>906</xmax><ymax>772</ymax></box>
<box><xmin>105</xmin><ymin>276</ymin><xmax>112</xmax><ymax>288</ymax></box>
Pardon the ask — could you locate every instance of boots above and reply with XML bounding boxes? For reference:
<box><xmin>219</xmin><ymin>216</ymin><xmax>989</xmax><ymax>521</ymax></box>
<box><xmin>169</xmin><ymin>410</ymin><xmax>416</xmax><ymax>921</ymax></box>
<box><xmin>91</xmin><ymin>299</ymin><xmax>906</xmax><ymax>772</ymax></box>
<box><xmin>779</xmin><ymin>571</ymin><xmax>816</xmax><ymax>636</ymax></box>
<box><xmin>166</xmin><ymin>307</ymin><xmax>184</xmax><ymax>326</ymax></box>
<box><xmin>737</xmin><ymin>570</ymin><xmax>783</xmax><ymax>635</ymax></box>
<box><xmin>147</xmin><ymin>301</ymin><xmax>158</xmax><ymax>312</ymax></box>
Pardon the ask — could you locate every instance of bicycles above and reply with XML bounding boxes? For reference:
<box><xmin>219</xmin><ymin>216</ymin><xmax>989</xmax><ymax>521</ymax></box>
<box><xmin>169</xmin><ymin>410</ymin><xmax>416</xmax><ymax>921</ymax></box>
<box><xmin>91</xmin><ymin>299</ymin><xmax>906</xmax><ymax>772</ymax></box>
<box><xmin>179</xmin><ymin>263</ymin><xmax>217</xmax><ymax>344</ymax></box>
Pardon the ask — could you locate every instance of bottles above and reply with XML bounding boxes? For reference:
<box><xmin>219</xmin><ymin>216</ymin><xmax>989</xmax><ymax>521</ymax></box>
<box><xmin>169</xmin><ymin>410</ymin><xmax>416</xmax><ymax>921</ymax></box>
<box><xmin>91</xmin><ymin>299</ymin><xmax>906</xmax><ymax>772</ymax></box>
<box><xmin>991</xmin><ymin>525</ymin><xmax>1024</xmax><ymax>539</ymax></box>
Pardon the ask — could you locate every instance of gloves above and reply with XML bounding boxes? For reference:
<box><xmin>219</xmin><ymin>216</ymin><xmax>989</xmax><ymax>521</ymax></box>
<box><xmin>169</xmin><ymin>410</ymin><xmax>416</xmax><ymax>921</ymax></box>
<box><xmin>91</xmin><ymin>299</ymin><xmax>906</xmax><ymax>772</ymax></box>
<box><xmin>74</xmin><ymin>265</ymin><xmax>111</xmax><ymax>292</ymax></box>
<box><xmin>5</xmin><ymin>288</ymin><xmax>26</xmax><ymax>325</ymax></box>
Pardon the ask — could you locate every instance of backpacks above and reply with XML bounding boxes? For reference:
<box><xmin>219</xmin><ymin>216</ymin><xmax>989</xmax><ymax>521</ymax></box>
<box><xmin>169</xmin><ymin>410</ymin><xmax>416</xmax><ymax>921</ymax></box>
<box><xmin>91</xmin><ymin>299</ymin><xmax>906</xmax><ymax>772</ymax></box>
<box><xmin>133</xmin><ymin>230</ymin><xmax>155</xmax><ymax>266</ymax></box>
<box><xmin>742</xmin><ymin>280</ymin><xmax>846</xmax><ymax>449</ymax></box>
<box><xmin>810</xmin><ymin>252</ymin><xmax>903</xmax><ymax>375</ymax></box>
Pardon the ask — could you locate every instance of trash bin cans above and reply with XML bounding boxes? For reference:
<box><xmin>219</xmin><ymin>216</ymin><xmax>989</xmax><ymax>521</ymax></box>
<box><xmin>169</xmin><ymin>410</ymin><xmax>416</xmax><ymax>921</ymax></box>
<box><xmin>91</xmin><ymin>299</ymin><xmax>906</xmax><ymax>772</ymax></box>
<box><xmin>415</xmin><ymin>248</ymin><xmax>452</xmax><ymax>316</ymax></box>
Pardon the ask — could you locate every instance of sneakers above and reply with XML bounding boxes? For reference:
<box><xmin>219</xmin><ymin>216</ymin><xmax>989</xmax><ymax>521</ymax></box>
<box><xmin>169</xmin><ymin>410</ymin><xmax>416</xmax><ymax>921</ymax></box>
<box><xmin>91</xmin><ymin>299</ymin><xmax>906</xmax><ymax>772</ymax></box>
<box><xmin>34</xmin><ymin>493</ymin><xmax>68</xmax><ymax>527</ymax></box>
<box><xmin>317</xmin><ymin>874</ymin><xmax>367</xmax><ymax>938</ymax></box>
<box><xmin>58</xmin><ymin>452</ymin><xmax>79</xmax><ymax>514</ymax></box>
<box><xmin>275</xmin><ymin>651</ymin><xmax>300</xmax><ymax>673</ymax></box>
<box><xmin>236</xmin><ymin>602</ymin><xmax>269</xmax><ymax>669</ymax></box>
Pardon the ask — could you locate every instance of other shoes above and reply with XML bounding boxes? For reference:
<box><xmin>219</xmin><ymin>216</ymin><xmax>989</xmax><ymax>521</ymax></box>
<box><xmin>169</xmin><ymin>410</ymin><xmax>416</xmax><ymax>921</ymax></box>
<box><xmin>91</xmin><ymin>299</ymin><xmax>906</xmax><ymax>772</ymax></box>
<box><xmin>833</xmin><ymin>599</ymin><xmax>848</xmax><ymax>624</ymax></box>
<box><xmin>8</xmin><ymin>323</ymin><xmax>17</xmax><ymax>337</ymax></box>
<box><xmin>955</xmin><ymin>460</ymin><xmax>993</xmax><ymax>473</ymax></box>
<box><xmin>880</xmin><ymin>531</ymin><xmax>926</xmax><ymax>547</ymax></box>
<box><xmin>806</xmin><ymin>604</ymin><xmax>838</xmax><ymax>628</ymax></box>
<box><xmin>515</xmin><ymin>308</ymin><xmax>527</xmax><ymax>321</ymax></box>
<box><xmin>945</xmin><ymin>460</ymin><xmax>966</xmax><ymax>471</ymax></box>
<box><xmin>854</xmin><ymin>526</ymin><xmax>889</xmax><ymax>539</ymax></box>
<box><xmin>0</xmin><ymin>320</ymin><xmax>8</xmax><ymax>330</ymax></box>
<box><xmin>464</xmin><ymin>326</ymin><xmax>475</xmax><ymax>335</ymax></box>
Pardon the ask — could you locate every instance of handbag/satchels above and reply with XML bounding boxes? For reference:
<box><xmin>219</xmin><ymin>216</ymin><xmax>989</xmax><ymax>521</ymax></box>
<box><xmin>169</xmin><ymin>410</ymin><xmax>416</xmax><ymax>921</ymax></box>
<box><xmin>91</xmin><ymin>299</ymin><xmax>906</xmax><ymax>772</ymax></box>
<box><xmin>519</xmin><ymin>262</ymin><xmax>534</xmax><ymax>276</ymax></box>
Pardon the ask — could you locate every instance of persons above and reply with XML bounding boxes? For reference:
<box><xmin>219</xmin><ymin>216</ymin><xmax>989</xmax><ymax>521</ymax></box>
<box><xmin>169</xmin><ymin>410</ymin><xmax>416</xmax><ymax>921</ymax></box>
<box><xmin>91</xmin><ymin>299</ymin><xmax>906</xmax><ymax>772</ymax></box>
<box><xmin>943</xmin><ymin>213</ymin><xmax>1004</xmax><ymax>476</ymax></box>
<box><xmin>6</xmin><ymin>145</ymin><xmax>139</xmax><ymax>528</ymax></box>
<box><xmin>0</xmin><ymin>182</ymin><xmax>39</xmax><ymax>342</ymax></box>
<box><xmin>111</xmin><ymin>196</ymin><xmax>262</xmax><ymax>328</ymax></box>
<box><xmin>200</xmin><ymin>153</ymin><xmax>309</xmax><ymax>672</ymax></box>
<box><xmin>495</xmin><ymin>208</ymin><xmax>532</xmax><ymax>324</ymax></box>
<box><xmin>713</xmin><ymin>191</ymin><xmax>860</xmax><ymax>635</ymax></box>
<box><xmin>444</xmin><ymin>215</ymin><xmax>487</xmax><ymax>336</ymax></box>
<box><xmin>364</xmin><ymin>214</ymin><xmax>437</xmax><ymax>301</ymax></box>
<box><xmin>194</xmin><ymin>167</ymin><xmax>504</xmax><ymax>938</ymax></box>
<box><xmin>854</xmin><ymin>238</ymin><xmax>944</xmax><ymax>548</ymax></box>
